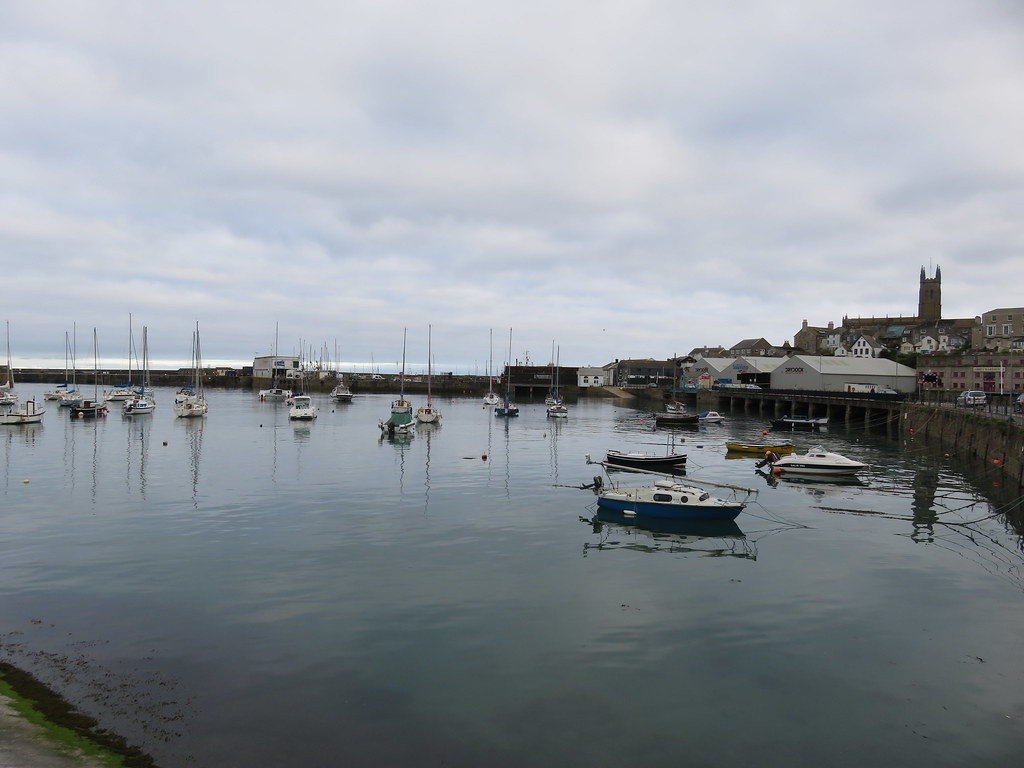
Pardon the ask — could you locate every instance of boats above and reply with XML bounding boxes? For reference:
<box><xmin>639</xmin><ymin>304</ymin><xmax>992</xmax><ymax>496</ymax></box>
<box><xmin>768</xmin><ymin>413</ymin><xmax>829</xmax><ymax>431</ymax></box>
<box><xmin>725</xmin><ymin>441</ymin><xmax>795</xmax><ymax>455</ymax></box>
<box><xmin>0</xmin><ymin>396</ymin><xmax>47</xmax><ymax>425</ymax></box>
<box><xmin>580</xmin><ymin>477</ymin><xmax>747</xmax><ymax>521</ymax></box>
<box><xmin>288</xmin><ymin>394</ymin><xmax>318</xmax><ymax>420</ymax></box>
<box><xmin>698</xmin><ymin>412</ymin><xmax>724</xmax><ymax>423</ymax></box>
<box><xmin>754</xmin><ymin>447</ymin><xmax>867</xmax><ymax>475</ymax></box>
<box><xmin>663</xmin><ymin>401</ymin><xmax>687</xmax><ymax>415</ymax></box>
<box><xmin>330</xmin><ymin>381</ymin><xmax>354</xmax><ymax>402</ymax></box>
<box><xmin>604</xmin><ymin>435</ymin><xmax>687</xmax><ymax>474</ymax></box>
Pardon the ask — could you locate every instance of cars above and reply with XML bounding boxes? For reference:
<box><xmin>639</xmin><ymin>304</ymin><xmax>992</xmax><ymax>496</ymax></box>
<box><xmin>685</xmin><ymin>384</ymin><xmax>695</xmax><ymax>389</ymax></box>
<box><xmin>648</xmin><ymin>383</ymin><xmax>657</xmax><ymax>388</ymax></box>
<box><xmin>745</xmin><ymin>385</ymin><xmax>762</xmax><ymax>389</ymax></box>
<box><xmin>957</xmin><ymin>390</ymin><xmax>988</xmax><ymax>409</ymax></box>
<box><xmin>877</xmin><ymin>388</ymin><xmax>898</xmax><ymax>395</ymax></box>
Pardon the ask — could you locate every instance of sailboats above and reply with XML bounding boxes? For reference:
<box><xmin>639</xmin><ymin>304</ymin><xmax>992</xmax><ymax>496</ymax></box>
<box><xmin>544</xmin><ymin>339</ymin><xmax>559</xmax><ymax>407</ymax></box>
<box><xmin>547</xmin><ymin>346</ymin><xmax>568</xmax><ymax>419</ymax></box>
<box><xmin>43</xmin><ymin>312</ymin><xmax>209</xmax><ymax>418</ymax></box>
<box><xmin>481</xmin><ymin>328</ymin><xmax>500</xmax><ymax>405</ymax></box>
<box><xmin>377</xmin><ymin>326</ymin><xmax>416</xmax><ymax>441</ymax></box>
<box><xmin>0</xmin><ymin>319</ymin><xmax>19</xmax><ymax>404</ymax></box>
<box><xmin>495</xmin><ymin>327</ymin><xmax>521</xmax><ymax>417</ymax></box>
<box><xmin>257</xmin><ymin>321</ymin><xmax>293</xmax><ymax>399</ymax></box>
<box><xmin>414</xmin><ymin>323</ymin><xmax>443</xmax><ymax>422</ymax></box>
